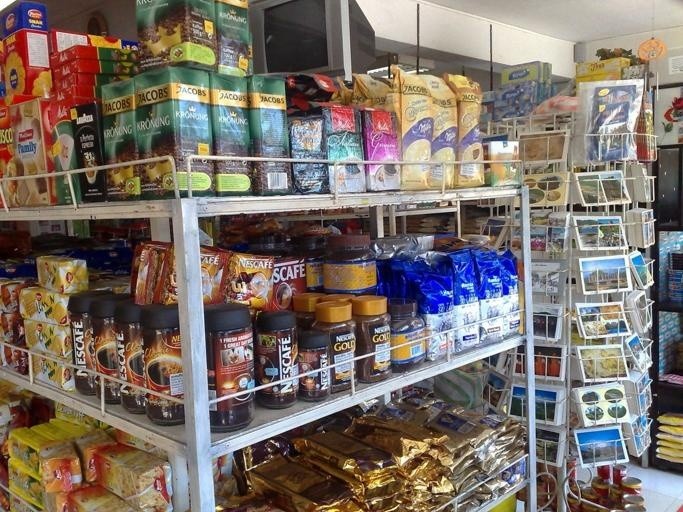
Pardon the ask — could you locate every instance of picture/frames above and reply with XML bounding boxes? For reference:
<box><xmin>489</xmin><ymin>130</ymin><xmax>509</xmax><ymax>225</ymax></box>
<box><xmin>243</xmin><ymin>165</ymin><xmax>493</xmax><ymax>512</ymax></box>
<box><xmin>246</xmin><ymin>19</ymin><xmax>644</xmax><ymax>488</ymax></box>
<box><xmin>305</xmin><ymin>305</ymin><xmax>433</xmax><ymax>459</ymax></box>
<box><xmin>650</xmin><ymin>82</ymin><xmax>683</xmax><ymax>146</ymax></box>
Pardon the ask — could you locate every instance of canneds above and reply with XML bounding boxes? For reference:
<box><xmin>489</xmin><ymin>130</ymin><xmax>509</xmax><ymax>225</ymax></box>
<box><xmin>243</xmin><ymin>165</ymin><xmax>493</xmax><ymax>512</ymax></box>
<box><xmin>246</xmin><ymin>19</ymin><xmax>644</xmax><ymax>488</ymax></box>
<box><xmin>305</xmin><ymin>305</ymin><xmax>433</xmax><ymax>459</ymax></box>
<box><xmin>567</xmin><ymin>465</ymin><xmax>646</xmax><ymax>512</ymax></box>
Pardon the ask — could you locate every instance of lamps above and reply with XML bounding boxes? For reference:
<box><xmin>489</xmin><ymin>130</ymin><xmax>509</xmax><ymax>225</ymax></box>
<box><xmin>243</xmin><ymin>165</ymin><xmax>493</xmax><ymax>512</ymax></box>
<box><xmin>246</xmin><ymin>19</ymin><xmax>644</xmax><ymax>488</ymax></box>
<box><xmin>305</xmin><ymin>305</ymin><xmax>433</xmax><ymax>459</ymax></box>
<box><xmin>367</xmin><ymin>54</ymin><xmax>435</xmax><ymax>79</ymax></box>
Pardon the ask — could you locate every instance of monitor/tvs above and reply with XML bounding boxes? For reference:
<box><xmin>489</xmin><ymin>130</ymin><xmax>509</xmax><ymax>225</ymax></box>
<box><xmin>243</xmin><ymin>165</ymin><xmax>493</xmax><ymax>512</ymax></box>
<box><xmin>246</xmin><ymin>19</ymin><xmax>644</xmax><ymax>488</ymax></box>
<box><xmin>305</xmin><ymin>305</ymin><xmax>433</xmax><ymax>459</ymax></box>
<box><xmin>249</xmin><ymin>0</ymin><xmax>376</xmax><ymax>86</ymax></box>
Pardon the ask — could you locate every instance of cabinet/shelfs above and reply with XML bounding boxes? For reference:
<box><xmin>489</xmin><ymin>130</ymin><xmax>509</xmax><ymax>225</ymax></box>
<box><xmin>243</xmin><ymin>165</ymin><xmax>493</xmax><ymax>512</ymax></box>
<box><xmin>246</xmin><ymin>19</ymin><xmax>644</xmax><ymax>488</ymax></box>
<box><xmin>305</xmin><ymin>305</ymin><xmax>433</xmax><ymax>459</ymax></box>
<box><xmin>649</xmin><ymin>145</ymin><xmax>683</xmax><ymax>474</ymax></box>
<box><xmin>0</xmin><ymin>155</ymin><xmax>537</xmax><ymax>512</ymax></box>
